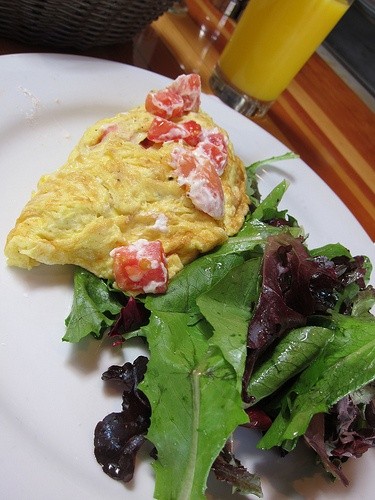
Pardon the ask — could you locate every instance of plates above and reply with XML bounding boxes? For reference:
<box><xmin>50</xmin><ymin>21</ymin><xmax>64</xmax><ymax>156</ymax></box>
<box><xmin>0</xmin><ymin>53</ymin><xmax>375</xmax><ymax>500</ymax></box>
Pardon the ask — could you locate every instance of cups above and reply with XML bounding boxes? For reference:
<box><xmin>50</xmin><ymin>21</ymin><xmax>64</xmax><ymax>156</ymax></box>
<box><xmin>208</xmin><ymin>0</ymin><xmax>354</xmax><ymax>119</ymax></box>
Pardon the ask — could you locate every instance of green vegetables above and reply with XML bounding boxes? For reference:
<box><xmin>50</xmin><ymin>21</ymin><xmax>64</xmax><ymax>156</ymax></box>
<box><xmin>65</xmin><ymin>151</ymin><xmax>375</xmax><ymax>500</ymax></box>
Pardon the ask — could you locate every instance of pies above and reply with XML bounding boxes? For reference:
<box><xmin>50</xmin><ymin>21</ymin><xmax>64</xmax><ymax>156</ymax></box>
<box><xmin>3</xmin><ymin>71</ymin><xmax>251</xmax><ymax>295</ymax></box>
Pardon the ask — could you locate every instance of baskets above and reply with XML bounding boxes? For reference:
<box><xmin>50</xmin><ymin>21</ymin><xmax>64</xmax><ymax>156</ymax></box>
<box><xmin>0</xmin><ymin>0</ymin><xmax>185</xmax><ymax>51</ymax></box>
